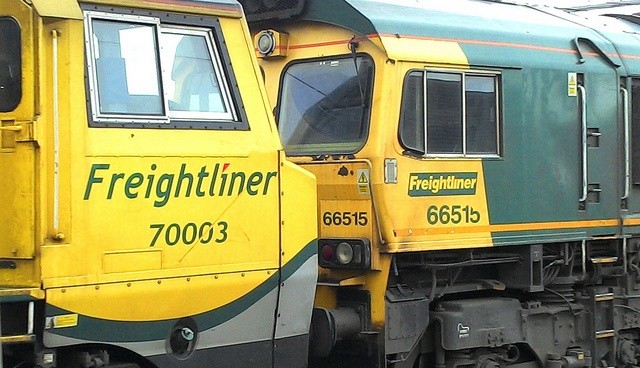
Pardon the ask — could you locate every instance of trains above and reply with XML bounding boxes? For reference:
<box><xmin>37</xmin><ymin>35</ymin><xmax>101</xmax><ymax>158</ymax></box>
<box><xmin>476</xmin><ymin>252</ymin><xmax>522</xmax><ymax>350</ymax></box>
<box><xmin>0</xmin><ymin>0</ymin><xmax>640</xmax><ymax>368</ymax></box>
